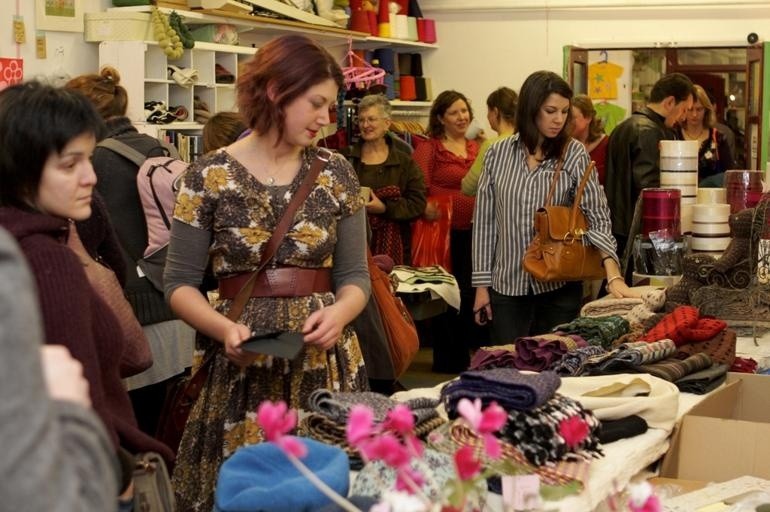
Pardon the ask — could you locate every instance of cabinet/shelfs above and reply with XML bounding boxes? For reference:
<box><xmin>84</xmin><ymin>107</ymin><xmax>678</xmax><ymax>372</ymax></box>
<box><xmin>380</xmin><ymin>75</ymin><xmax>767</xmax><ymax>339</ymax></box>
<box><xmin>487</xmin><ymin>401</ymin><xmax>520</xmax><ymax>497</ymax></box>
<box><xmin>96</xmin><ymin>4</ymin><xmax>439</xmax><ymax>161</ymax></box>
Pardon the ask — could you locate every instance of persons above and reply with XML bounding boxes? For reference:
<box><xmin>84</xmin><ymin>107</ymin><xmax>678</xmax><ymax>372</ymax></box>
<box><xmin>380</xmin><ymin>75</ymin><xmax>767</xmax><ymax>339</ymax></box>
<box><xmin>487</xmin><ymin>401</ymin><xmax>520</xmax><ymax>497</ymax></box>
<box><xmin>0</xmin><ymin>78</ymin><xmax>179</xmax><ymax>509</ymax></box>
<box><xmin>163</xmin><ymin>33</ymin><xmax>377</xmax><ymax>512</ymax></box>
<box><xmin>0</xmin><ymin>225</ymin><xmax>123</xmax><ymax>509</ymax></box>
<box><xmin>350</xmin><ymin>69</ymin><xmax>739</xmax><ymax>374</ymax></box>
<box><xmin>203</xmin><ymin>111</ymin><xmax>247</xmax><ymax>153</ymax></box>
<box><xmin>65</xmin><ymin>68</ymin><xmax>197</xmax><ymax>443</ymax></box>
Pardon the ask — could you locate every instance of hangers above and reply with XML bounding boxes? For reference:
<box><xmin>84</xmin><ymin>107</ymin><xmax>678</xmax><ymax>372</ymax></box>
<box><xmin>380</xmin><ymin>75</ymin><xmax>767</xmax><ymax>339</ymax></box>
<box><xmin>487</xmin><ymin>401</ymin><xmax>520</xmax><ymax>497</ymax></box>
<box><xmin>587</xmin><ymin>52</ymin><xmax>624</xmax><ymax>70</ymax></box>
<box><xmin>589</xmin><ymin>99</ymin><xmax>621</xmax><ymax>110</ymax></box>
<box><xmin>390</xmin><ymin>114</ymin><xmax>429</xmax><ymax>136</ymax></box>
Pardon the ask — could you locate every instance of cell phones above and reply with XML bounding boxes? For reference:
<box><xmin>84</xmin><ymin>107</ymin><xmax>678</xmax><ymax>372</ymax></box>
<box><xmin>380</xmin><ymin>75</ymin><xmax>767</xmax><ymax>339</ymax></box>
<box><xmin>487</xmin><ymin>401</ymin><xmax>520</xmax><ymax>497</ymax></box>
<box><xmin>360</xmin><ymin>187</ymin><xmax>370</xmax><ymax>205</ymax></box>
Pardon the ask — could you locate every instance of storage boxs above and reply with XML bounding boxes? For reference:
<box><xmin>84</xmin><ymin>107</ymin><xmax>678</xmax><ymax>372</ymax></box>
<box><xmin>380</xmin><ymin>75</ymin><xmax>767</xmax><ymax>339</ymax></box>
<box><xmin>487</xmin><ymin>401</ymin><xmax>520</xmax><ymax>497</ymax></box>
<box><xmin>83</xmin><ymin>11</ymin><xmax>171</xmax><ymax>43</ymax></box>
<box><xmin>661</xmin><ymin>368</ymin><xmax>770</xmax><ymax>481</ymax></box>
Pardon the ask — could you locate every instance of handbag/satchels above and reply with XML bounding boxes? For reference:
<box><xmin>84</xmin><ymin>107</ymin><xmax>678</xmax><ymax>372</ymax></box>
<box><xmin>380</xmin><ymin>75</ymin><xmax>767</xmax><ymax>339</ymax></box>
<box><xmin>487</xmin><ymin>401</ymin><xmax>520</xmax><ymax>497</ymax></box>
<box><xmin>62</xmin><ymin>238</ymin><xmax>154</xmax><ymax>379</ymax></box>
<box><xmin>157</xmin><ymin>375</ymin><xmax>197</xmax><ymax>450</ymax></box>
<box><xmin>525</xmin><ymin>204</ymin><xmax>607</xmax><ymax>281</ymax></box>
<box><xmin>367</xmin><ymin>265</ymin><xmax>417</xmax><ymax>380</ymax></box>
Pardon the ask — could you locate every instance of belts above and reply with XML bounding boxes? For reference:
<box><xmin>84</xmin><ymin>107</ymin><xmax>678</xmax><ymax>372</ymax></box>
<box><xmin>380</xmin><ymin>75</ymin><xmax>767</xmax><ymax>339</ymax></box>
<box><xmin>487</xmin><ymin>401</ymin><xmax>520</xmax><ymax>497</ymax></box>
<box><xmin>219</xmin><ymin>267</ymin><xmax>335</xmax><ymax>299</ymax></box>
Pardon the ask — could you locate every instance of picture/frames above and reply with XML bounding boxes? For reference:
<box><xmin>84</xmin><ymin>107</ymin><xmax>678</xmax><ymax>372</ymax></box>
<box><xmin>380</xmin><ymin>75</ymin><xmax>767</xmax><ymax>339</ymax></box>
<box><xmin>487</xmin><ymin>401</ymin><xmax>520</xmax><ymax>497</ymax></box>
<box><xmin>34</xmin><ymin>0</ymin><xmax>85</xmax><ymax>34</ymax></box>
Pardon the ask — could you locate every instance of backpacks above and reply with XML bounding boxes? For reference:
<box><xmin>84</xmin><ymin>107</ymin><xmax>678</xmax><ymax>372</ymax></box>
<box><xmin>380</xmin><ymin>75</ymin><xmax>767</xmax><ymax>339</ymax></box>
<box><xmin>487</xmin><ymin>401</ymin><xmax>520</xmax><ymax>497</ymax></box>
<box><xmin>97</xmin><ymin>137</ymin><xmax>218</xmax><ymax>293</ymax></box>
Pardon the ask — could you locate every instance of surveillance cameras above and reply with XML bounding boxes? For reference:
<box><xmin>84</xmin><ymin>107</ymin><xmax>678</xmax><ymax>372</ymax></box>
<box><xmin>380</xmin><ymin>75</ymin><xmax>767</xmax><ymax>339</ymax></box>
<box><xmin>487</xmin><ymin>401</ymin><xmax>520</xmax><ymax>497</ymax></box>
<box><xmin>747</xmin><ymin>32</ymin><xmax>758</xmax><ymax>44</ymax></box>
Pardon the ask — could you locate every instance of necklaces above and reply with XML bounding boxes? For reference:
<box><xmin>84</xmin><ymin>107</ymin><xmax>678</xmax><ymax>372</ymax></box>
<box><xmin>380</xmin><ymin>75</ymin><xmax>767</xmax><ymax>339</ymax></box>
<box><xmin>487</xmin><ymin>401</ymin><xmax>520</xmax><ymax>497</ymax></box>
<box><xmin>250</xmin><ymin>146</ymin><xmax>297</xmax><ymax>186</ymax></box>
